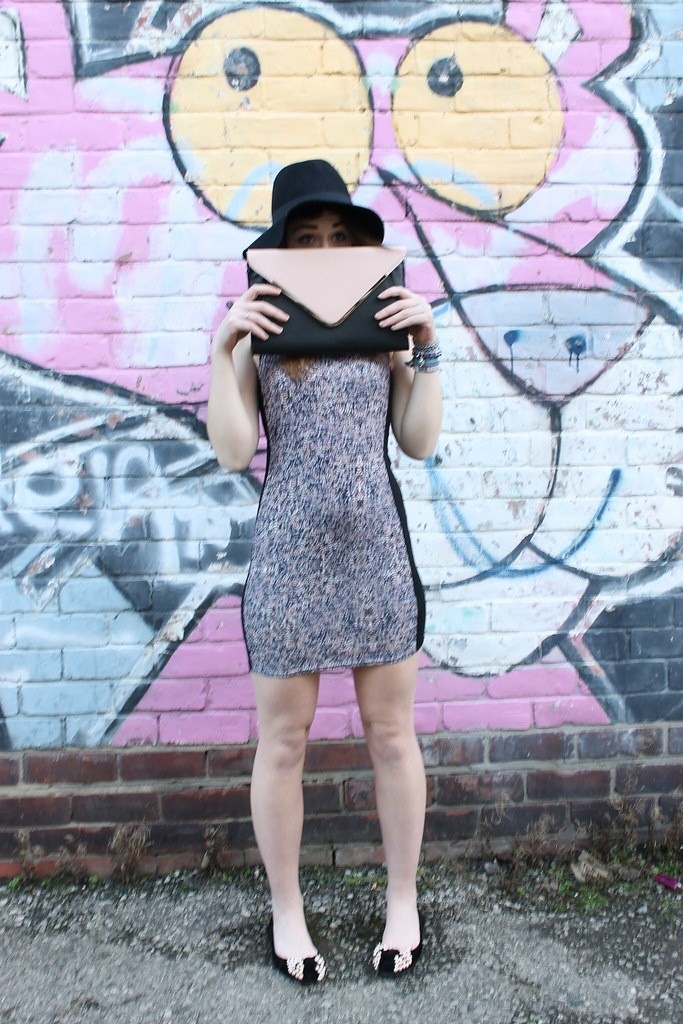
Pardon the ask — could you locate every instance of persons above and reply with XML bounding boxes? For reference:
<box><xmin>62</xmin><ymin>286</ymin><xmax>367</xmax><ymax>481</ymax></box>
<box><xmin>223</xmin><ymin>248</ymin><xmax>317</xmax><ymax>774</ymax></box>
<box><xmin>204</xmin><ymin>161</ymin><xmax>443</xmax><ymax>987</ymax></box>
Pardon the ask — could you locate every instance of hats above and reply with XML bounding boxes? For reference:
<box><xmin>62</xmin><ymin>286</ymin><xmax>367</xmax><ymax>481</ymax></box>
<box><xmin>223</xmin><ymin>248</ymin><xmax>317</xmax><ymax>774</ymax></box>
<box><xmin>242</xmin><ymin>159</ymin><xmax>385</xmax><ymax>259</ymax></box>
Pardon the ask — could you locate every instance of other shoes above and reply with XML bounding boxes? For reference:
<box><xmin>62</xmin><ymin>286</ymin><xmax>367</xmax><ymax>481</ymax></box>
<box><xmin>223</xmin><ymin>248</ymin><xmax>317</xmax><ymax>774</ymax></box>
<box><xmin>374</xmin><ymin>915</ymin><xmax>426</xmax><ymax>976</ymax></box>
<box><xmin>267</xmin><ymin>915</ymin><xmax>327</xmax><ymax>983</ymax></box>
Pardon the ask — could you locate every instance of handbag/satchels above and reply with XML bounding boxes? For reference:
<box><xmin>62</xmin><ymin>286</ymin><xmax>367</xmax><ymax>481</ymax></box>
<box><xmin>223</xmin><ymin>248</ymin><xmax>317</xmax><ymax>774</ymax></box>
<box><xmin>246</xmin><ymin>246</ymin><xmax>409</xmax><ymax>355</ymax></box>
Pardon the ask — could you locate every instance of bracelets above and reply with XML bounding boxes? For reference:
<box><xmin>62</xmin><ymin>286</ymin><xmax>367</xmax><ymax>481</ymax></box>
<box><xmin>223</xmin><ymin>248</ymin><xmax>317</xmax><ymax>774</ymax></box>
<box><xmin>404</xmin><ymin>337</ymin><xmax>441</xmax><ymax>373</ymax></box>
